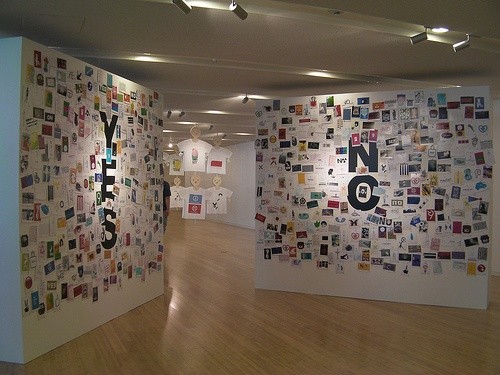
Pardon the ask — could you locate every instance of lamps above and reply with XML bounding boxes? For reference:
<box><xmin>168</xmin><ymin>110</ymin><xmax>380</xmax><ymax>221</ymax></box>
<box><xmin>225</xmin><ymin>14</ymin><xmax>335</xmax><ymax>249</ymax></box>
<box><xmin>166</xmin><ymin>110</ymin><xmax>171</xmax><ymax>118</ymax></box>
<box><xmin>172</xmin><ymin>0</ymin><xmax>192</xmax><ymax>15</ymax></box>
<box><xmin>410</xmin><ymin>26</ymin><xmax>433</xmax><ymax>45</ymax></box>
<box><xmin>242</xmin><ymin>94</ymin><xmax>249</xmax><ymax>105</ymax></box>
<box><xmin>179</xmin><ymin>112</ymin><xmax>185</xmax><ymax>117</ymax></box>
<box><xmin>229</xmin><ymin>0</ymin><xmax>248</xmax><ymax>20</ymax></box>
<box><xmin>453</xmin><ymin>34</ymin><xmax>471</xmax><ymax>53</ymax></box>
